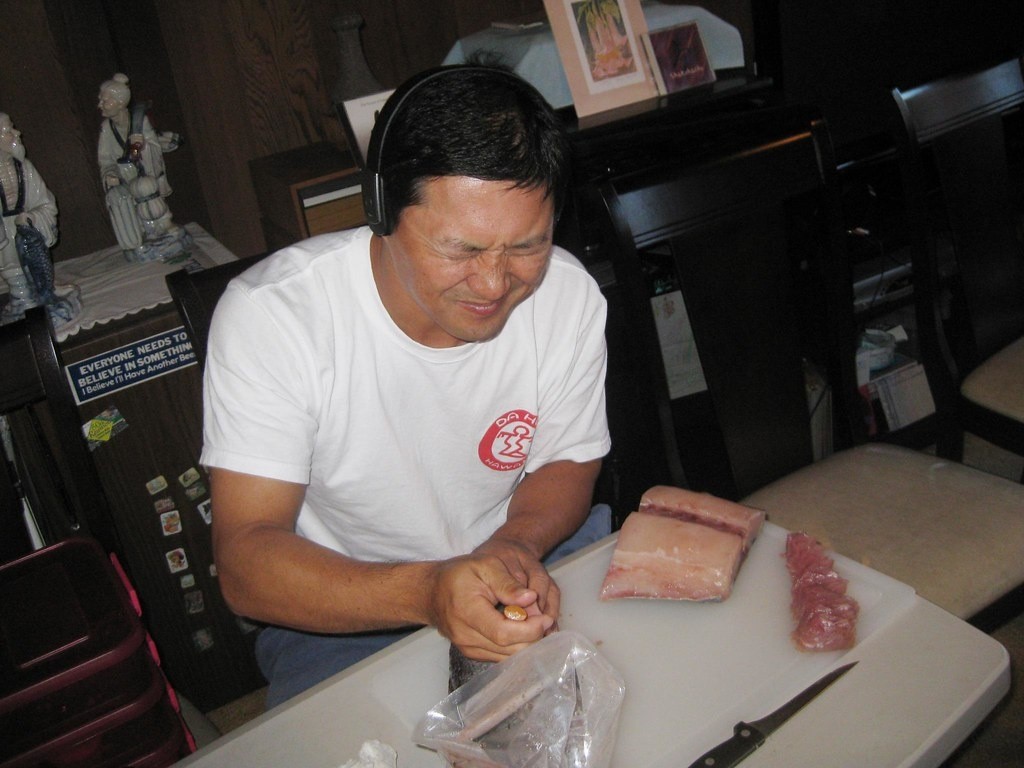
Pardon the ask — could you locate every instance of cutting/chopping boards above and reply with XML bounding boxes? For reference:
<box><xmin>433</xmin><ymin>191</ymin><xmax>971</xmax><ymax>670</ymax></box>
<box><xmin>180</xmin><ymin>521</ymin><xmax>914</xmax><ymax>767</ymax></box>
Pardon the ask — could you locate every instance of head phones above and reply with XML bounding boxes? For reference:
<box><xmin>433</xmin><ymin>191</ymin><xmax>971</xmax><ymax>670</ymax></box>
<box><xmin>360</xmin><ymin>62</ymin><xmax>569</xmax><ymax>237</ymax></box>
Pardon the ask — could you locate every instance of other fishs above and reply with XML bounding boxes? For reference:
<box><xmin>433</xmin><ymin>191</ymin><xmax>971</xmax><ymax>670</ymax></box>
<box><xmin>448</xmin><ymin>593</ymin><xmax>589</xmax><ymax>768</ymax></box>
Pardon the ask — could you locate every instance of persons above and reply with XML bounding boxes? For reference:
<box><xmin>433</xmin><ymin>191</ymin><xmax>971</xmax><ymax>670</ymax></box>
<box><xmin>98</xmin><ymin>74</ymin><xmax>173</xmax><ymax>240</ymax></box>
<box><xmin>0</xmin><ymin>112</ymin><xmax>58</xmax><ymax>312</ymax></box>
<box><xmin>200</xmin><ymin>67</ymin><xmax>612</xmax><ymax>707</ymax></box>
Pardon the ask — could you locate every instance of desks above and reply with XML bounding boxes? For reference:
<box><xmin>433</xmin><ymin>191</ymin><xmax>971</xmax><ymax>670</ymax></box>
<box><xmin>170</xmin><ymin>336</ymin><xmax>1024</xmax><ymax>768</ymax></box>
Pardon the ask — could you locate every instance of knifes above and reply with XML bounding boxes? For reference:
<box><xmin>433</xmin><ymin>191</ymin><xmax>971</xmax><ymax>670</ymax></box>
<box><xmin>687</xmin><ymin>660</ymin><xmax>861</xmax><ymax>767</ymax></box>
